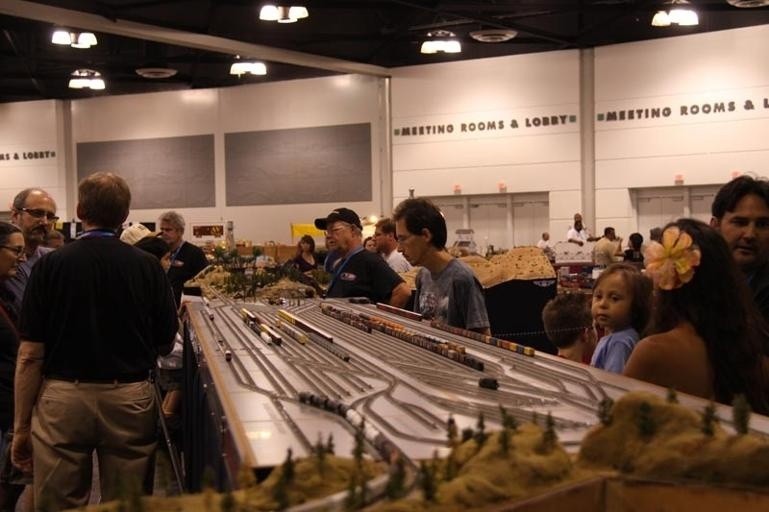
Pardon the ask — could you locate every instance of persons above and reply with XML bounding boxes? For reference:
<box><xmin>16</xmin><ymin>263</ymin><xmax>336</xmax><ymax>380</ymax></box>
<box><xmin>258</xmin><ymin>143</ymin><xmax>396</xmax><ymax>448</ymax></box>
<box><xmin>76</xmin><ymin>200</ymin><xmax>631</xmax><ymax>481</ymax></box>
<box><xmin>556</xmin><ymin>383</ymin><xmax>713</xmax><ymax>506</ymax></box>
<box><xmin>138</xmin><ymin>237</ymin><xmax>172</xmax><ymax>273</ymax></box>
<box><xmin>706</xmin><ymin>174</ymin><xmax>769</xmax><ymax>389</ymax></box>
<box><xmin>121</xmin><ymin>222</ymin><xmax>164</xmax><ymax>249</ymax></box>
<box><xmin>585</xmin><ymin>259</ymin><xmax>654</xmax><ymax>376</ymax></box>
<box><xmin>540</xmin><ymin>289</ymin><xmax>600</xmax><ymax>364</ymax></box>
<box><xmin>618</xmin><ymin>216</ymin><xmax>767</xmax><ymax>417</ymax></box>
<box><xmin>391</xmin><ymin>197</ymin><xmax>493</xmax><ymax>336</ymax></box>
<box><xmin>11</xmin><ymin>167</ymin><xmax>182</xmax><ymax>512</ymax></box>
<box><xmin>45</xmin><ymin>228</ymin><xmax>63</xmax><ymax>248</ymax></box>
<box><xmin>0</xmin><ymin>221</ymin><xmax>28</xmax><ymax>431</ymax></box>
<box><xmin>5</xmin><ymin>187</ymin><xmax>59</xmax><ymax>304</ymax></box>
<box><xmin>157</xmin><ymin>209</ymin><xmax>204</xmax><ymax>276</ymax></box>
<box><xmin>312</xmin><ymin>206</ymin><xmax>413</xmax><ymax>311</ymax></box>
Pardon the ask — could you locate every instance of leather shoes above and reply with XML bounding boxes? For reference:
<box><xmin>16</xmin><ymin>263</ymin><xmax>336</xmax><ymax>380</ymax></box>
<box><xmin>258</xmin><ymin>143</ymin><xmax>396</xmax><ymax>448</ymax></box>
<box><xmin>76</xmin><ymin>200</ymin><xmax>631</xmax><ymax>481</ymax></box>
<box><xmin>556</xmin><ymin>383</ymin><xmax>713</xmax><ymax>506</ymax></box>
<box><xmin>44</xmin><ymin>370</ymin><xmax>150</xmax><ymax>385</ymax></box>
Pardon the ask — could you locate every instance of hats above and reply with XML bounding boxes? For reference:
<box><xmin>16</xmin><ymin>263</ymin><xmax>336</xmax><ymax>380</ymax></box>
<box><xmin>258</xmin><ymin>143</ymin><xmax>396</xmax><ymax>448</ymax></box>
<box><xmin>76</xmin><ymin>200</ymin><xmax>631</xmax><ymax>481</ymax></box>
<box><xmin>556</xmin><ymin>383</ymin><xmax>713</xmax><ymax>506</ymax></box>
<box><xmin>314</xmin><ymin>208</ymin><xmax>363</xmax><ymax>230</ymax></box>
<box><xmin>118</xmin><ymin>222</ymin><xmax>171</xmax><ymax>261</ymax></box>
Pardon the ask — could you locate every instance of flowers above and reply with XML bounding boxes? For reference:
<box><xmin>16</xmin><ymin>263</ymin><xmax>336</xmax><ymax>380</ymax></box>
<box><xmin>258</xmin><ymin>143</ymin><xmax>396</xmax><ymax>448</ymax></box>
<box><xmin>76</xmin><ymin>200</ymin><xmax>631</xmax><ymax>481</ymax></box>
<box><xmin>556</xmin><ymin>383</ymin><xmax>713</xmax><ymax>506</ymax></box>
<box><xmin>639</xmin><ymin>226</ymin><xmax>702</xmax><ymax>291</ymax></box>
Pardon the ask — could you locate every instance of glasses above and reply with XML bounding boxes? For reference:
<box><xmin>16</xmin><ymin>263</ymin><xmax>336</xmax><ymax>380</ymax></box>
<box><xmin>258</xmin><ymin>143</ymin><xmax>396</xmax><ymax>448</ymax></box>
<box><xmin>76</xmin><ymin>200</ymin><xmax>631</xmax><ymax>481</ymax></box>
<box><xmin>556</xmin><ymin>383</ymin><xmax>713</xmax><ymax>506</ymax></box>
<box><xmin>14</xmin><ymin>206</ymin><xmax>59</xmax><ymax>223</ymax></box>
<box><xmin>395</xmin><ymin>232</ymin><xmax>416</xmax><ymax>246</ymax></box>
<box><xmin>323</xmin><ymin>226</ymin><xmax>351</xmax><ymax>237</ymax></box>
<box><xmin>0</xmin><ymin>244</ymin><xmax>26</xmax><ymax>256</ymax></box>
